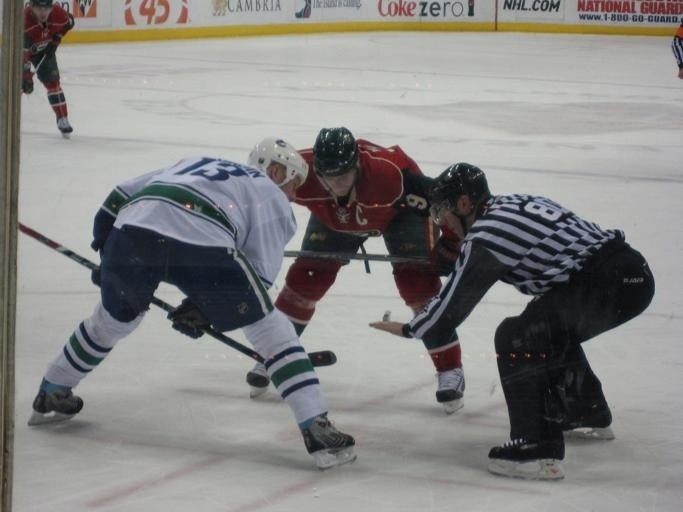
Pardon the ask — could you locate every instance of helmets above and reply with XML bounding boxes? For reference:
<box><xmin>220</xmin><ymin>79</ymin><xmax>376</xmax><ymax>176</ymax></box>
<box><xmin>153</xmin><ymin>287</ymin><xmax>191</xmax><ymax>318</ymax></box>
<box><xmin>428</xmin><ymin>163</ymin><xmax>492</xmax><ymax>223</ymax></box>
<box><xmin>312</xmin><ymin>127</ymin><xmax>359</xmax><ymax>177</ymax></box>
<box><xmin>247</xmin><ymin>134</ymin><xmax>309</xmax><ymax>190</ymax></box>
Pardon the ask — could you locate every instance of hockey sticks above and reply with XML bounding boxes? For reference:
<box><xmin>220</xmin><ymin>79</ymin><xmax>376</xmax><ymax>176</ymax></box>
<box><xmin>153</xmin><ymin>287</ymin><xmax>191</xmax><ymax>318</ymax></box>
<box><xmin>17</xmin><ymin>222</ymin><xmax>336</xmax><ymax>368</ymax></box>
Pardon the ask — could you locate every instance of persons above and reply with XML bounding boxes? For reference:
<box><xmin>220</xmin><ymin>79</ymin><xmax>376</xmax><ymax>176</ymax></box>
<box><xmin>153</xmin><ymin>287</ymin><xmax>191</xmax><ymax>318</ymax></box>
<box><xmin>27</xmin><ymin>133</ymin><xmax>358</xmax><ymax>455</ymax></box>
<box><xmin>246</xmin><ymin>126</ymin><xmax>466</xmax><ymax>406</ymax></box>
<box><xmin>671</xmin><ymin>22</ymin><xmax>683</xmax><ymax>81</ymax></box>
<box><xmin>23</xmin><ymin>0</ymin><xmax>76</xmax><ymax>139</ymax></box>
<box><xmin>371</xmin><ymin>161</ymin><xmax>657</xmax><ymax>465</ymax></box>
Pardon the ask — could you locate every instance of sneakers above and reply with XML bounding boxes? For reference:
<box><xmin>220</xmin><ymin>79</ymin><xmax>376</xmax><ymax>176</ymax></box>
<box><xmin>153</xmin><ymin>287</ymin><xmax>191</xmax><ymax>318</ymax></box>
<box><xmin>299</xmin><ymin>413</ymin><xmax>355</xmax><ymax>453</ymax></box>
<box><xmin>33</xmin><ymin>388</ymin><xmax>84</xmax><ymax>414</ymax></box>
<box><xmin>489</xmin><ymin>435</ymin><xmax>565</xmax><ymax>464</ymax></box>
<box><xmin>246</xmin><ymin>362</ymin><xmax>269</xmax><ymax>388</ymax></box>
<box><xmin>435</xmin><ymin>367</ymin><xmax>466</xmax><ymax>402</ymax></box>
<box><xmin>57</xmin><ymin>118</ymin><xmax>73</xmax><ymax>133</ymax></box>
<box><xmin>561</xmin><ymin>403</ymin><xmax>612</xmax><ymax>431</ymax></box>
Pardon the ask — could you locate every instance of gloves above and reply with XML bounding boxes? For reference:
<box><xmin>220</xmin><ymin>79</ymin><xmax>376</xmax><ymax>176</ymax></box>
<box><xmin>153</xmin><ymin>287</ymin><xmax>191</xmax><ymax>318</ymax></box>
<box><xmin>167</xmin><ymin>299</ymin><xmax>208</xmax><ymax>339</ymax></box>
<box><xmin>427</xmin><ymin>232</ymin><xmax>461</xmax><ymax>278</ymax></box>
